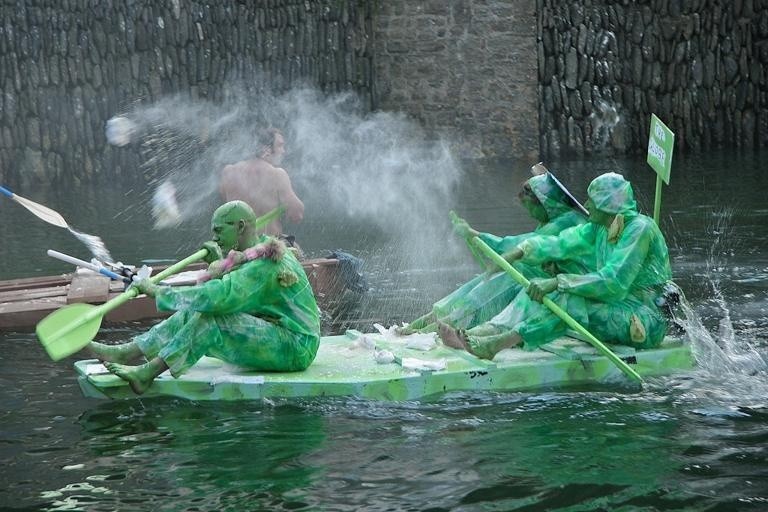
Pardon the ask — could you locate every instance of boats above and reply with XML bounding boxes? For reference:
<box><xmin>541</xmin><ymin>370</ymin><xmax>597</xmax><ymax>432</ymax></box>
<box><xmin>0</xmin><ymin>251</ymin><xmax>369</xmax><ymax>332</ymax></box>
<box><xmin>73</xmin><ymin>332</ymin><xmax>704</xmax><ymax>402</ymax></box>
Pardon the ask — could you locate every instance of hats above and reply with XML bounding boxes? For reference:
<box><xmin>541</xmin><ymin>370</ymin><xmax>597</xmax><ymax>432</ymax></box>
<box><xmin>586</xmin><ymin>172</ymin><xmax>639</xmax><ymax>217</ymax></box>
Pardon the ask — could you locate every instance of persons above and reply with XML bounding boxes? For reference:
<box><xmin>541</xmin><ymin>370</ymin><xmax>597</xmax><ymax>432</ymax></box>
<box><xmin>221</xmin><ymin>129</ymin><xmax>306</xmax><ymax>260</ymax></box>
<box><xmin>435</xmin><ymin>173</ymin><xmax>672</xmax><ymax>360</ymax></box>
<box><xmin>85</xmin><ymin>200</ymin><xmax>319</xmax><ymax>394</ymax></box>
<box><xmin>396</xmin><ymin>174</ymin><xmax>588</xmax><ymax>333</ymax></box>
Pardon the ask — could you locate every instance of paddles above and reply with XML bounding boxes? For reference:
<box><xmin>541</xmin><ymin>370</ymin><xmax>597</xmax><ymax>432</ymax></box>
<box><xmin>33</xmin><ymin>205</ymin><xmax>284</xmax><ymax>362</ymax></box>
<box><xmin>470</xmin><ymin>236</ymin><xmax>646</xmax><ymax>384</ymax></box>
<box><xmin>2</xmin><ymin>187</ymin><xmax>68</xmax><ymax>230</ymax></box>
